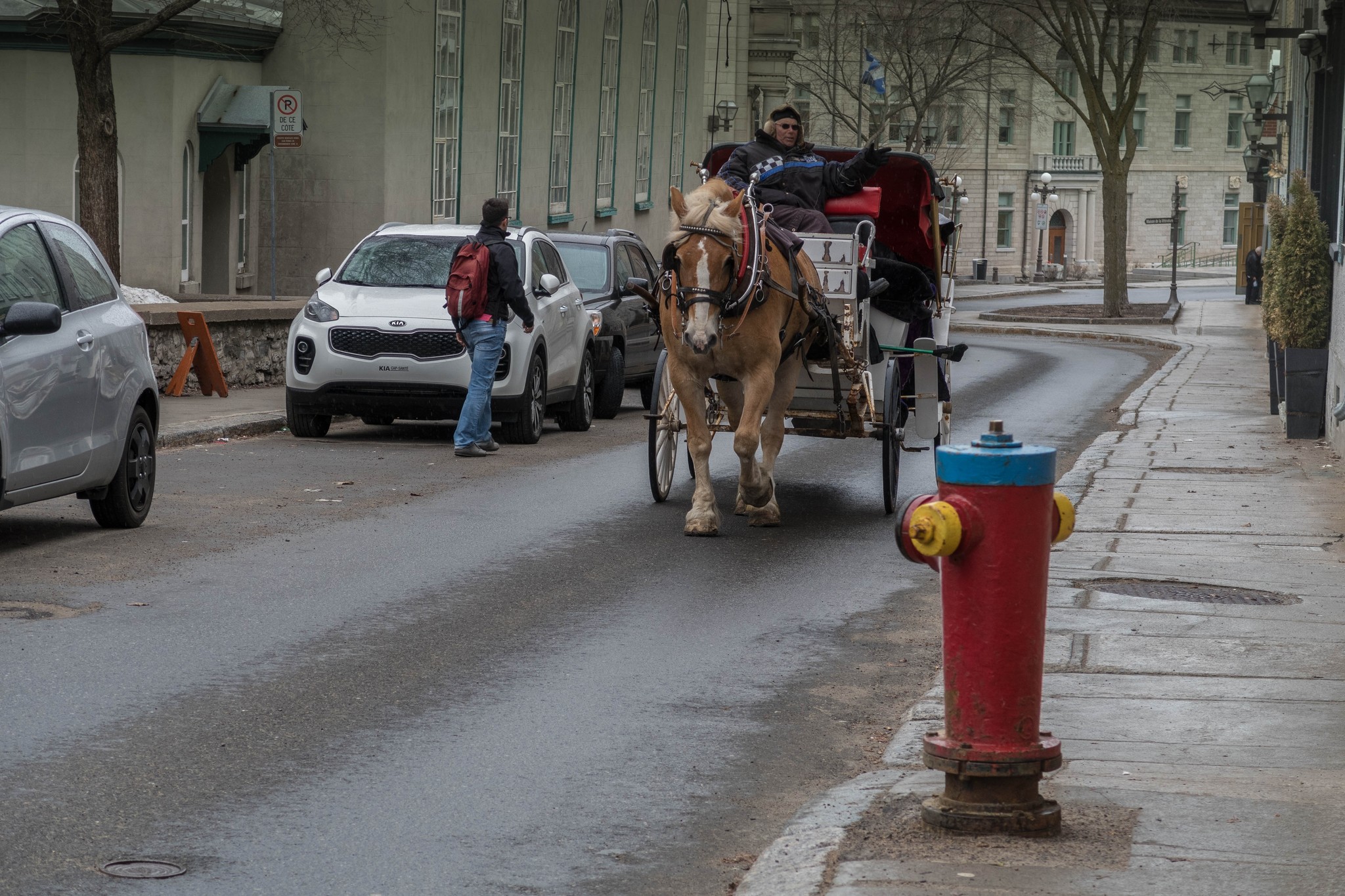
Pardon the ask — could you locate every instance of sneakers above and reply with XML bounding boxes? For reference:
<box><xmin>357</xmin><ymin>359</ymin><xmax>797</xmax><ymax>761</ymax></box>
<box><xmin>477</xmin><ymin>440</ymin><xmax>500</xmax><ymax>452</ymax></box>
<box><xmin>455</xmin><ymin>441</ymin><xmax>487</xmax><ymax>457</ymax></box>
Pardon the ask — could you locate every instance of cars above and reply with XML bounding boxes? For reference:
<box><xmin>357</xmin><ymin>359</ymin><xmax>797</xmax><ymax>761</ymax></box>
<box><xmin>0</xmin><ymin>202</ymin><xmax>163</xmax><ymax>530</ymax></box>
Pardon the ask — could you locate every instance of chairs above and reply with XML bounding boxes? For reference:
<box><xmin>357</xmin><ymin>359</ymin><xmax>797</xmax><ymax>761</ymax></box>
<box><xmin>13</xmin><ymin>253</ymin><xmax>40</xmax><ymax>288</ymax></box>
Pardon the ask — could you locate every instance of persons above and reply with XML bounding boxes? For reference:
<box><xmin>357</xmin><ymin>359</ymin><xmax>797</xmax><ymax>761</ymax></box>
<box><xmin>444</xmin><ymin>197</ymin><xmax>534</xmax><ymax>458</ymax></box>
<box><xmin>1245</xmin><ymin>246</ymin><xmax>1263</xmax><ymax>305</ymax></box>
<box><xmin>716</xmin><ymin>104</ymin><xmax>892</xmax><ymax>233</ymax></box>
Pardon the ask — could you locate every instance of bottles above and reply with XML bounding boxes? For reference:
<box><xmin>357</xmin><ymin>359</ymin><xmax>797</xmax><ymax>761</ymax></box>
<box><xmin>1253</xmin><ymin>278</ymin><xmax>1258</xmax><ymax>288</ymax></box>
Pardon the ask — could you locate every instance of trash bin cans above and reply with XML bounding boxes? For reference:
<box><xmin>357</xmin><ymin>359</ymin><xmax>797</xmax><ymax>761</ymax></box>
<box><xmin>973</xmin><ymin>258</ymin><xmax>987</xmax><ymax>279</ymax></box>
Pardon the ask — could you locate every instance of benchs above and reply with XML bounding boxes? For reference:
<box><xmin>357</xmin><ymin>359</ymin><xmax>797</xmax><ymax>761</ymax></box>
<box><xmin>825</xmin><ymin>184</ymin><xmax>883</xmax><ymax>242</ymax></box>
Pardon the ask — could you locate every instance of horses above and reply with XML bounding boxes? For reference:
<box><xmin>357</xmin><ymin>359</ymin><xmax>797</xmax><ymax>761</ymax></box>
<box><xmin>658</xmin><ymin>179</ymin><xmax>824</xmax><ymax>536</ymax></box>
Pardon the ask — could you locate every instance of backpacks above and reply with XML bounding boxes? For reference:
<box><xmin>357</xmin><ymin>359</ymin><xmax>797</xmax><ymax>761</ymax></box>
<box><xmin>445</xmin><ymin>235</ymin><xmax>516</xmax><ymax>319</ymax></box>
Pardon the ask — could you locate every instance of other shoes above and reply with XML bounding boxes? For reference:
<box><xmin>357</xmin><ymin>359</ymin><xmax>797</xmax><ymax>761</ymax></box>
<box><xmin>1249</xmin><ymin>301</ymin><xmax>1261</xmax><ymax>305</ymax></box>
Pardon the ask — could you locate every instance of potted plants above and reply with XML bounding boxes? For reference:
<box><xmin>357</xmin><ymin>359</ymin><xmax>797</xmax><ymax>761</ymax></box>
<box><xmin>1261</xmin><ymin>175</ymin><xmax>1332</xmax><ymax>439</ymax></box>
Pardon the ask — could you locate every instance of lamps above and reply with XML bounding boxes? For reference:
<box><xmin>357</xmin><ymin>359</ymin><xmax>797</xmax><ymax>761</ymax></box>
<box><xmin>1242</xmin><ymin>72</ymin><xmax>1293</xmax><ymax>182</ymax></box>
<box><xmin>707</xmin><ymin>101</ymin><xmax>738</xmax><ymax>131</ymax></box>
<box><xmin>1244</xmin><ymin>0</ymin><xmax>1313</xmax><ymax>51</ymax></box>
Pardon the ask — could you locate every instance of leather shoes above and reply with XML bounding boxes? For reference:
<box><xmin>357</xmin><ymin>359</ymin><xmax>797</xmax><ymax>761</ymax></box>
<box><xmin>868</xmin><ymin>278</ymin><xmax>889</xmax><ymax>298</ymax></box>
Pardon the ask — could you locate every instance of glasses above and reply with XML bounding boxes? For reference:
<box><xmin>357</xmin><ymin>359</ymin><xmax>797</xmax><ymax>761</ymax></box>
<box><xmin>775</xmin><ymin>122</ymin><xmax>800</xmax><ymax>130</ymax></box>
<box><xmin>503</xmin><ymin>215</ymin><xmax>511</xmax><ymax>221</ymax></box>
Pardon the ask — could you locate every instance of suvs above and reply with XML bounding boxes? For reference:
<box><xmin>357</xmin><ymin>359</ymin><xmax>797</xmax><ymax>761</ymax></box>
<box><xmin>539</xmin><ymin>229</ymin><xmax>666</xmax><ymax>421</ymax></box>
<box><xmin>284</xmin><ymin>221</ymin><xmax>598</xmax><ymax>444</ymax></box>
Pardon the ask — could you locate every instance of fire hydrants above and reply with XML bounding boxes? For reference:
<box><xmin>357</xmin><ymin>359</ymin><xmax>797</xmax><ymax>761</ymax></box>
<box><xmin>889</xmin><ymin>418</ymin><xmax>1078</xmax><ymax>835</ymax></box>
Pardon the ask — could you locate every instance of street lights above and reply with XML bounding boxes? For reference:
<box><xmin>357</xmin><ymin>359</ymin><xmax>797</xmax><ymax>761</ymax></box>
<box><xmin>946</xmin><ymin>176</ymin><xmax>968</xmax><ymax>277</ymax></box>
<box><xmin>1030</xmin><ymin>172</ymin><xmax>1058</xmax><ymax>282</ymax></box>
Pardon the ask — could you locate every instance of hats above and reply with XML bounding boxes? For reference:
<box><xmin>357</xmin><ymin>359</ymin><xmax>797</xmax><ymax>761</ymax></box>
<box><xmin>763</xmin><ymin>102</ymin><xmax>805</xmax><ymax>147</ymax></box>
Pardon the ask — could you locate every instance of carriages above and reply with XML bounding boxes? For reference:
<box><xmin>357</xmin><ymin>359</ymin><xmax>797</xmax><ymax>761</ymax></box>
<box><xmin>624</xmin><ymin>133</ymin><xmax>968</xmax><ymax>511</ymax></box>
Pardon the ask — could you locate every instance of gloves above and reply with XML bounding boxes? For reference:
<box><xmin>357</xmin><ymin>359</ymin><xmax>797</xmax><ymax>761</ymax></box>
<box><xmin>866</xmin><ymin>142</ymin><xmax>892</xmax><ymax>167</ymax></box>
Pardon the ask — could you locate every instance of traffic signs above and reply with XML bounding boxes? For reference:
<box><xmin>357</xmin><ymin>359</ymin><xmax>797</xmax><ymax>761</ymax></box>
<box><xmin>1143</xmin><ymin>218</ymin><xmax>1174</xmax><ymax>224</ymax></box>
